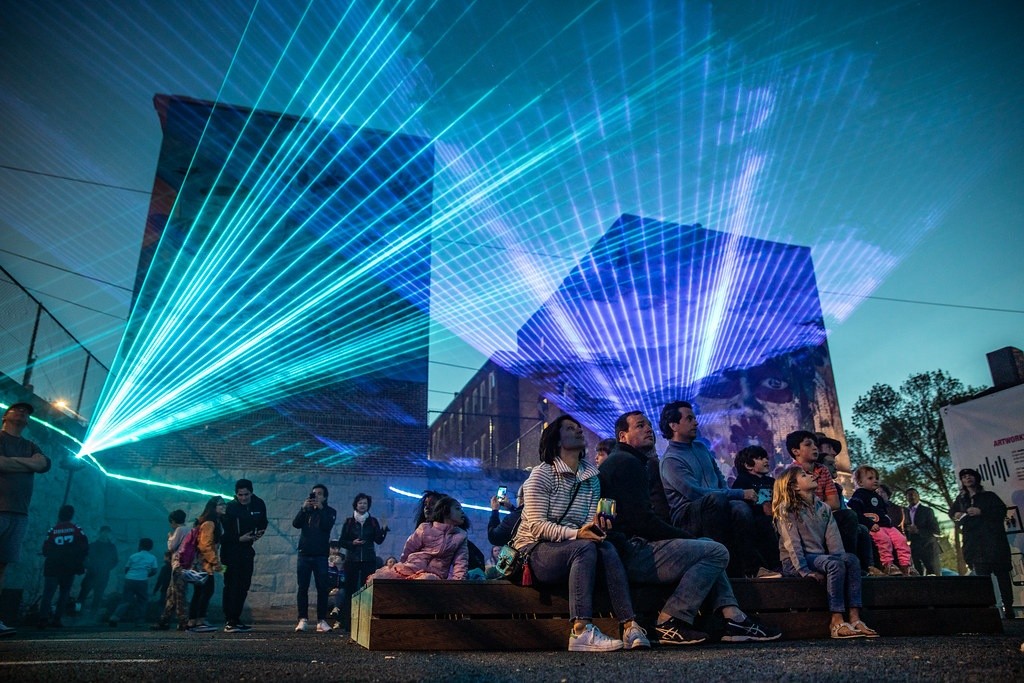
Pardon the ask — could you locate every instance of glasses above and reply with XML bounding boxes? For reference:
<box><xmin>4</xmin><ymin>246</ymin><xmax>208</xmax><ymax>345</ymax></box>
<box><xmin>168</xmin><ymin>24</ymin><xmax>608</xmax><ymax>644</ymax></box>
<box><xmin>961</xmin><ymin>474</ymin><xmax>971</xmax><ymax>477</ymax></box>
<box><xmin>514</xmin><ymin>494</ymin><xmax>522</xmax><ymax>498</ymax></box>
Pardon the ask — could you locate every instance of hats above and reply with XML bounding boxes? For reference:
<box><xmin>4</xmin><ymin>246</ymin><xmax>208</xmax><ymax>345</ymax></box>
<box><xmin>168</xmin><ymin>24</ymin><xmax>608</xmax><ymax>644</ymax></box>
<box><xmin>814</xmin><ymin>431</ymin><xmax>842</xmax><ymax>457</ymax></box>
<box><xmin>959</xmin><ymin>469</ymin><xmax>982</xmax><ymax>481</ymax></box>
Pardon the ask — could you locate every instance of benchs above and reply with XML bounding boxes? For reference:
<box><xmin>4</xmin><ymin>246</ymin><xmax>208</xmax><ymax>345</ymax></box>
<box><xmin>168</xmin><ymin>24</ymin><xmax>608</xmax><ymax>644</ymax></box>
<box><xmin>352</xmin><ymin>575</ymin><xmax>1005</xmax><ymax>651</ymax></box>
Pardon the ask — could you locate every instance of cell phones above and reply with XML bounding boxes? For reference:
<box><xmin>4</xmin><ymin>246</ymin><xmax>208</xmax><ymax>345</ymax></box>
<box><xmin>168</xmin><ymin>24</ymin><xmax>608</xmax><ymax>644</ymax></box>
<box><xmin>212</xmin><ymin>564</ymin><xmax>227</xmax><ymax>573</ymax></box>
<box><xmin>359</xmin><ymin>537</ymin><xmax>367</xmax><ymax>542</ymax></box>
<box><xmin>588</xmin><ymin>524</ymin><xmax>605</xmax><ymax>537</ymax></box>
<box><xmin>497</xmin><ymin>486</ymin><xmax>508</xmax><ymax>505</ymax></box>
<box><xmin>250</xmin><ymin>527</ymin><xmax>258</xmax><ymax>536</ymax></box>
<box><xmin>309</xmin><ymin>493</ymin><xmax>315</xmax><ymax>502</ymax></box>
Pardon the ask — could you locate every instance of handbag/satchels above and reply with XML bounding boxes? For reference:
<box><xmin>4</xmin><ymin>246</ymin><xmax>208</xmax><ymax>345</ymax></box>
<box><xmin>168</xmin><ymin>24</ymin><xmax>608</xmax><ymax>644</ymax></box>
<box><xmin>496</xmin><ymin>545</ymin><xmax>534</xmax><ymax>587</ymax></box>
<box><xmin>181</xmin><ymin>569</ymin><xmax>208</xmax><ymax>585</ymax></box>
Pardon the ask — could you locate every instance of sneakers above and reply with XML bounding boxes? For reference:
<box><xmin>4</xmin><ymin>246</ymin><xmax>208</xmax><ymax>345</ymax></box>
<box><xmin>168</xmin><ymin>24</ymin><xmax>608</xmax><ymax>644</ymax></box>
<box><xmin>623</xmin><ymin>621</ymin><xmax>651</xmax><ymax>651</ymax></box>
<box><xmin>224</xmin><ymin>623</ymin><xmax>241</xmax><ymax>633</ymax></box>
<box><xmin>654</xmin><ymin>617</ymin><xmax>709</xmax><ymax>644</ymax></box>
<box><xmin>316</xmin><ymin>621</ymin><xmax>333</xmax><ymax>633</ymax></box>
<box><xmin>568</xmin><ymin>624</ymin><xmax>623</xmax><ymax>652</ymax></box>
<box><xmin>720</xmin><ymin>616</ymin><xmax>783</xmax><ymax>642</ymax></box>
<box><xmin>236</xmin><ymin>623</ymin><xmax>252</xmax><ymax>632</ymax></box>
<box><xmin>294</xmin><ymin>618</ymin><xmax>309</xmax><ymax>632</ymax></box>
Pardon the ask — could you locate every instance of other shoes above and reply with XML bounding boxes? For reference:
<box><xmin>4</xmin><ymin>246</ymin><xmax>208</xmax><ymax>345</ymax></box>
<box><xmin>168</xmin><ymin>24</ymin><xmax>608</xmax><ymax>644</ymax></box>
<box><xmin>195</xmin><ymin>624</ymin><xmax>218</xmax><ymax>632</ymax></box>
<box><xmin>332</xmin><ymin>607</ymin><xmax>340</xmax><ymax>616</ymax></box>
<box><xmin>176</xmin><ymin>624</ymin><xmax>184</xmax><ymax>631</ymax></box>
<box><xmin>745</xmin><ymin>567</ymin><xmax>784</xmax><ymax>579</ymax></box>
<box><xmin>108</xmin><ymin>619</ymin><xmax>117</xmax><ymax>628</ymax></box>
<box><xmin>861</xmin><ymin>571</ymin><xmax>870</xmax><ymax>577</ymax></box>
<box><xmin>882</xmin><ymin>564</ymin><xmax>903</xmax><ymax>576</ymax></box>
<box><xmin>1005</xmin><ymin>609</ymin><xmax>1017</xmax><ymax>619</ymax></box>
<box><xmin>0</xmin><ymin>620</ymin><xmax>17</xmax><ymax>638</ymax></box>
<box><xmin>185</xmin><ymin>625</ymin><xmax>195</xmax><ymax>632</ymax></box>
<box><xmin>869</xmin><ymin>565</ymin><xmax>889</xmax><ymax>577</ymax></box>
<box><xmin>900</xmin><ymin>565</ymin><xmax>921</xmax><ymax>577</ymax></box>
<box><xmin>333</xmin><ymin>621</ymin><xmax>342</xmax><ymax>629</ymax></box>
<box><xmin>151</xmin><ymin>624</ymin><xmax>170</xmax><ymax>631</ymax></box>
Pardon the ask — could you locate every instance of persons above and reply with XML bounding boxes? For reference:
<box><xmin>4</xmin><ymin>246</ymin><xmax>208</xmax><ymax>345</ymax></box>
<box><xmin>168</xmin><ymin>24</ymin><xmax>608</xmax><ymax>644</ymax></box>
<box><xmin>185</xmin><ymin>496</ymin><xmax>226</xmax><ymax>632</ymax></box>
<box><xmin>339</xmin><ymin>493</ymin><xmax>391</xmax><ymax>635</ymax></box>
<box><xmin>487</xmin><ymin>483</ymin><xmax>528</xmax><ymax>587</ymax></box>
<box><xmin>150</xmin><ymin>509</ymin><xmax>190</xmax><ymax>631</ymax></box>
<box><xmin>594</xmin><ymin>438</ymin><xmax>616</xmax><ymax>469</ymax></box>
<box><xmin>109</xmin><ymin>538</ymin><xmax>158</xmax><ymax>627</ymax></box>
<box><xmin>366</xmin><ymin>485</ymin><xmax>485</xmax><ymax>581</ymax></box>
<box><xmin>486</xmin><ymin>545</ymin><xmax>501</xmax><ymax>568</ymax></box>
<box><xmin>76</xmin><ymin>526</ymin><xmax>119</xmax><ymax>620</ymax></box>
<box><xmin>659</xmin><ymin>400</ymin><xmax>782</xmax><ymax>578</ymax></box>
<box><xmin>772</xmin><ymin>467</ymin><xmax>880</xmax><ymax>638</ymax></box>
<box><xmin>40</xmin><ymin>504</ymin><xmax>89</xmax><ymax>627</ymax></box>
<box><xmin>329</xmin><ymin>541</ymin><xmax>345</xmax><ymax>629</ymax></box>
<box><xmin>152</xmin><ymin>552</ymin><xmax>172</xmax><ymax>601</ymax></box>
<box><xmin>385</xmin><ymin>556</ymin><xmax>398</xmax><ymax>568</ymax></box>
<box><xmin>508</xmin><ymin>414</ymin><xmax>651</xmax><ymax>652</ymax></box>
<box><xmin>220</xmin><ymin>479</ymin><xmax>269</xmax><ymax>632</ymax></box>
<box><xmin>731</xmin><ymin>430</ymin><xmax>940</xmax><ymax>576</ymax></box>
<box><xmin>597</xmin><ymin>411</ymin><xmax>782</xmax><ymax>644</ymax></box>
<box><xmin>0</xmin><ymin>402</ymin><xmax>52</xmax><ymax>636</ymax></box>
<box><xmin>292</xmin><ymin>484</ymin><xmax>337</xmax><ymax>633</ymax></box>
<box><xmin>949</xmin><ymin>469</ymin><xmax>1017</xmax><ymax>634</ymax></box>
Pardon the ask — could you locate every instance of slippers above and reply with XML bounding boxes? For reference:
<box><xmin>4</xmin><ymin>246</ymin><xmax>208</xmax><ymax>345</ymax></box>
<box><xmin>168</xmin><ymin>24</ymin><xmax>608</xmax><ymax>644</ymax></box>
<box><xmin>831</xmin><ymin>622</ymin><xmax>866</xmax><ymax>638</ymax></box>
<box><xmin>852</xmin><ymin>620</ymin><xmax>881</xmax><ymax>638</ymax></box>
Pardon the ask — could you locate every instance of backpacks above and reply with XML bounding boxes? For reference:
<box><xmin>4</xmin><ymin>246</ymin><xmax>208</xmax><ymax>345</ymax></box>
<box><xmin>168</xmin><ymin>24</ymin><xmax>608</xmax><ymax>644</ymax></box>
<box><xmin>178</xmin><ymin>520</ymin><xmax>206</xmax><ymax>570</ymax></box>
